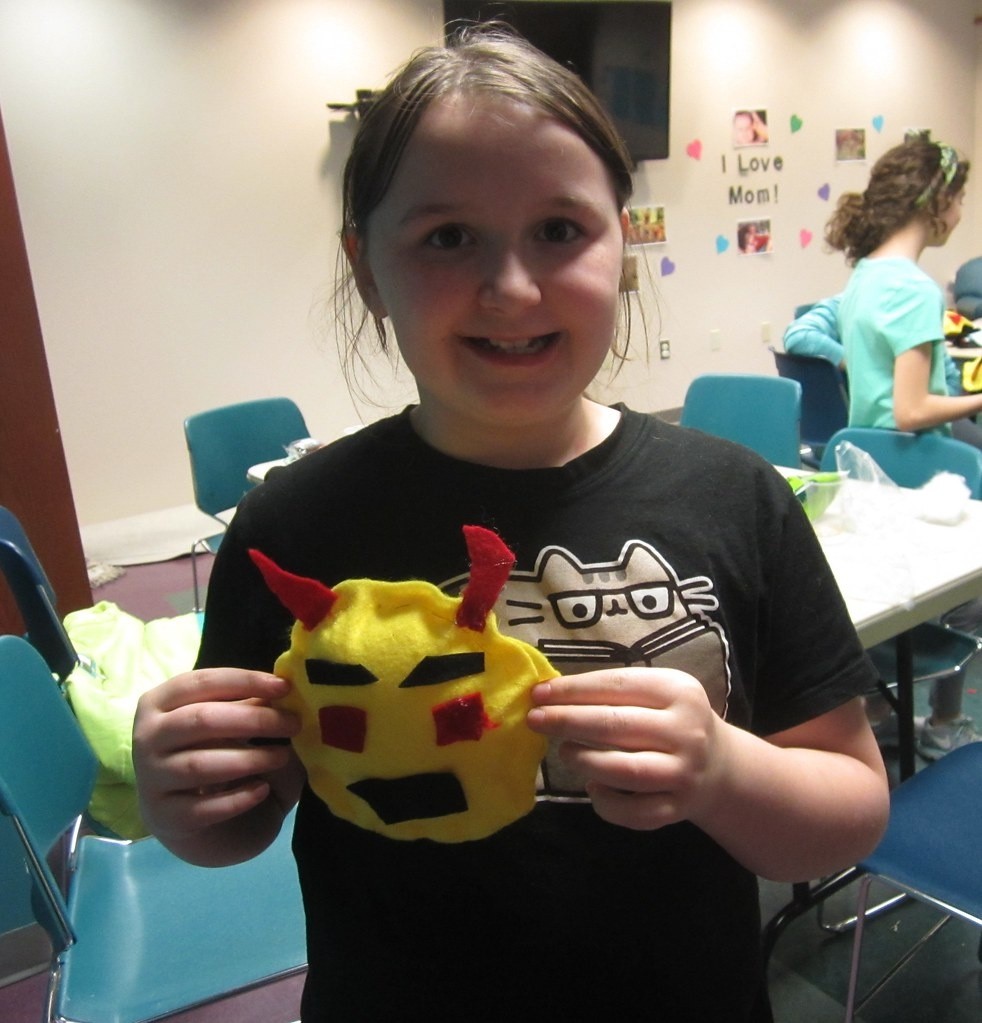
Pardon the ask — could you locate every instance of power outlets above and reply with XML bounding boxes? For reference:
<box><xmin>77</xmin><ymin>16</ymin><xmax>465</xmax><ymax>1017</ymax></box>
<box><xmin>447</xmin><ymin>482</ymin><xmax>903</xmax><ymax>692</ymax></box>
<box><xmin>661</xmin><ymin>340</ymin><xmax>670</xmax><ymax>360</ymax></box>
<box><xmin>709</xmin><ymin>329</ymin><xmax>721</xmax><ymax>350</ymax></box>
<box><xmin>761</xmin><ymin>322</ymin><xmax>772</xmax><ymax>344</ymax></box>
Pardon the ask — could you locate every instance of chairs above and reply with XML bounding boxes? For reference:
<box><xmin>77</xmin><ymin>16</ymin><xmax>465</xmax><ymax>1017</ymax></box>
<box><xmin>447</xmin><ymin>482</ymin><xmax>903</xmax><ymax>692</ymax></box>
<box><xmin>679</xmin><ymin>374</ymin><xmax>805</xmax><ymax>470</ymax></box>
<box><xmin>840</xmin><ymin>741</ymin><xmax>982</xmax><ymax>1023</ymax></box>
<box><xmin>0</xmin><ymin>502</ymin><xmax>202</xmax><ymax>841</ymax></box>
<box><xmin>0</xmin><ymin>627</ymin><xmax>308</xmax><ymax>1023</ymax></box>
<box><xmin>769</xmin><ymin>346</ymin><xmax>849</xmax><ymax>470</ymax></box>
<box><xmin>184</xmin><ymin>398</ymin><xmax>310</xmax><ymax>612</ymax></box>
<box><xmin>819</xmin><ymin>425</ymin><xmax>982</xmax><ymax>502</ymax></box>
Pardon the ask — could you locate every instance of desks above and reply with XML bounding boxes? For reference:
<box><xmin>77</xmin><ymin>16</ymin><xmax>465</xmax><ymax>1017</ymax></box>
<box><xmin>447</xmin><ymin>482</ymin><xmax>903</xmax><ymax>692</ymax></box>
<box><xmin>215</xmin><ymin>478</ymin><xmax>982</xmax><ymax>969</ymax></box>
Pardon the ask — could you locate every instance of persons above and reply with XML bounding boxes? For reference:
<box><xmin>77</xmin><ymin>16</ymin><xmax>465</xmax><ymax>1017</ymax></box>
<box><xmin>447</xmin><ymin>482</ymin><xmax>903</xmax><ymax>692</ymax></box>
<box><xmin>738</xmin><ymin>222</ymin><xmax>772</xmax><ymax>254</ymax></box>
<box><xmin>784</xmin><ymin>132</ymin><xmax>982</xmax><ymax>761</ymax></box>
<box><xmin>131</xmin><ymin>35</ymin><xmax>888</xmax><ymax>1022</ymax></box>
<box><xmin>734</xmin><ymin>111</ymin><xmax>767</xmax><ymax>145</ymax></box>
<box><xmin>625</xmin><ymin>210</ymin><xmax>664</xmax><ymax>243</ymax></box>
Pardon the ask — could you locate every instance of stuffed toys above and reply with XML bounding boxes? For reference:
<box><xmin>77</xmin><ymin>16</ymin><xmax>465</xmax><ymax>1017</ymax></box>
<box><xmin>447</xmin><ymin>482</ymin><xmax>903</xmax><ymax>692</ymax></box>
<box><xmin>248</xmin><ymin>524</ymin><xmax>564</xmax><ymax>845</ymax></box>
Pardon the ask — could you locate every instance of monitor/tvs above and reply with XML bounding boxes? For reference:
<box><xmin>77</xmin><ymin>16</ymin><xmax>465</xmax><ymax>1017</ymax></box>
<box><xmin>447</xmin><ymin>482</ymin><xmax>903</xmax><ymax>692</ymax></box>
<box><xmin>442</xmin><ymin>0</ymin><xmax>672</xmax><ymax>159</ymax></box>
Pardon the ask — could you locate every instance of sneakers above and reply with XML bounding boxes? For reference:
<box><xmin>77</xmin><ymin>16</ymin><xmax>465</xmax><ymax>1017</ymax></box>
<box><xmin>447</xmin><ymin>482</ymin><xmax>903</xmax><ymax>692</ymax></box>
<box><xmin>917</xmin><ymin>709</ymin><xmax>982</xmax><ymax>760</ymax></box>
<box><xmin>873</xmin><ymin>710</ymin><xmax>925</xmax><ymax>749</ymax></box>
